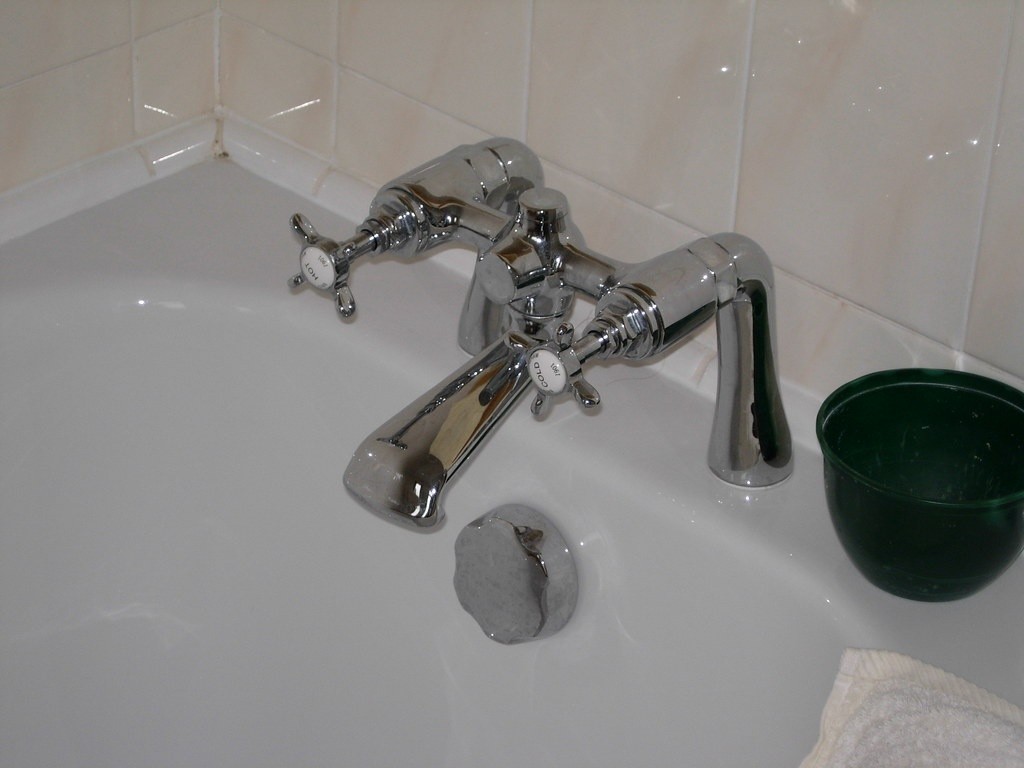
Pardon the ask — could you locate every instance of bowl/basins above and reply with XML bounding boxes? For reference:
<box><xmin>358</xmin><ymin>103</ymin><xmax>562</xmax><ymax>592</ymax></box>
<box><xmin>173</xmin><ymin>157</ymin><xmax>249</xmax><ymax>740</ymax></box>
<box><xmin>816</xmin><ymin>369</ymin><xmax>1024</xmax><ymax>602</ymax></box>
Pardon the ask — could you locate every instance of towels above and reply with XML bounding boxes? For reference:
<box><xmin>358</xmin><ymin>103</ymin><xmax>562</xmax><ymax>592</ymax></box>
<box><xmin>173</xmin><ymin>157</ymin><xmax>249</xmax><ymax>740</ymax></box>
<box><xmin>801</xmin><ymin>645</ymin><xmax>1023</xmax><ymax>768</ymax></box>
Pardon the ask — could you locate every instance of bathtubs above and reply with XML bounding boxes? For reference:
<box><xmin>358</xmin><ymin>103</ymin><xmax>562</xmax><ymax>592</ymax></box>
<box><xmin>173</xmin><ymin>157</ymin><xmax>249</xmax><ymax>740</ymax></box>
<box><xmin>0</xmin><ymin>154</ymin><xmax>1023</xmax><ymax>768</ymax></box>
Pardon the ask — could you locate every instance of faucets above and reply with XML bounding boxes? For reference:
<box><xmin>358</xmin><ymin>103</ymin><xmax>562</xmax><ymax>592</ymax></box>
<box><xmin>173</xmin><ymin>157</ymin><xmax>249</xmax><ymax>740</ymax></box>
<box><xmin>285</xmin><ymin>133</ymin><xmax>798</xmax><ymax>530</ymax></box>
